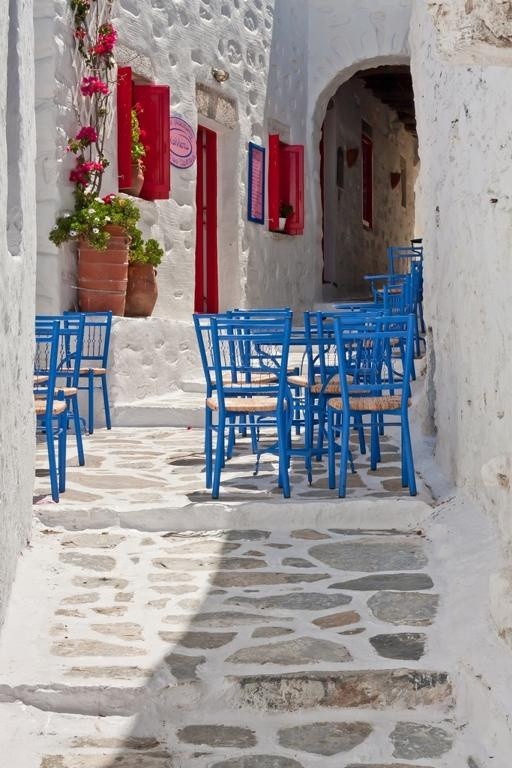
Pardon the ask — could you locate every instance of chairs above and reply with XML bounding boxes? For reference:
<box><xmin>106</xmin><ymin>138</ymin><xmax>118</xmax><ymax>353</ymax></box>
<box><xmin>34</xmin><ymin>312</ymin><xmax>112</xmax><ymax>503</ymax></box>
<box><xmin>191</xmin><ymin>243</ymin><xmax>423</xmax><ymax>502</ymax></box>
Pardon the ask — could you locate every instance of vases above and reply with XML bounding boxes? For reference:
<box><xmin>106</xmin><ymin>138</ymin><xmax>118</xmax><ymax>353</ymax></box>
<box><xmin>127</xmin><ymin>164</ymin><xmax>146</xmax><ymax>195</ymax></box>
<box><xmin>75</xmin><ymin>227</ymin><xmax>128</xmax><ymax>316</ymax></box>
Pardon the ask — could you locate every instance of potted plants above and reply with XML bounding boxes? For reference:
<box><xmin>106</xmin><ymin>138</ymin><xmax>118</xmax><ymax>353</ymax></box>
<box><xmin>278</xmin><ymin>201</ymin><xmax>293</xmax><ymax>231</ymax></box>
<box><xmin>128</xmin><ymin>231</ymin><xmax>164</xmax><ymax>317</ymax></box>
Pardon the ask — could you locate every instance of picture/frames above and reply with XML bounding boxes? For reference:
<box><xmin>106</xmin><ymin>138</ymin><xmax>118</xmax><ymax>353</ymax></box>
<box><xmin>247</xmin><ymin>142</ymin><xmax>265</xmax><ymax>225</ymax></box>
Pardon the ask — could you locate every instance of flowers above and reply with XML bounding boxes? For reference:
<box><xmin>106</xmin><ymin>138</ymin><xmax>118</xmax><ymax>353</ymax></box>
<box><xmin>130</xmin><ymin>103</ymin><xmax>152</xmax><ymax>161</ymax></box>
<box><xmin>50</xmin><ymin>1</ymin><xmax>132</xmax><ymax>247</ymax></box>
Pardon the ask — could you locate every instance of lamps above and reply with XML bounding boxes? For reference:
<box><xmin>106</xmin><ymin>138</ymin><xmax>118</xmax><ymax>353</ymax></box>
<box><xmin>211</xmin><ymin>66</ymin><xmax>229</xmax><ymax>84</ymax></box>
